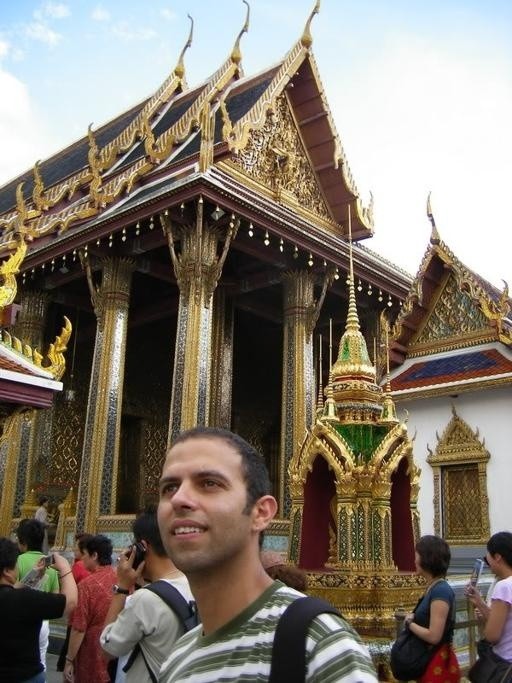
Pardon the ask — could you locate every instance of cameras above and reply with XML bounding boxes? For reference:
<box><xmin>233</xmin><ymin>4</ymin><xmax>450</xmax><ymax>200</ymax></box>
<box><xmin>43</xmin><ymin>554</ymin><xmax>56</xmax><ymax>567</ymax></box>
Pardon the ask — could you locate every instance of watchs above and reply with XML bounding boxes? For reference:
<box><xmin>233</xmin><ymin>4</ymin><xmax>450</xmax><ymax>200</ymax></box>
<box><xmin>111</xmin><ymin>584</ymin><xmax>129</xmax><ymax>594</ymax></box>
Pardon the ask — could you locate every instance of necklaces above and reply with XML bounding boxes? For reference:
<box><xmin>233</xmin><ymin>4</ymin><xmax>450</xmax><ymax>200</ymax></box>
<box><xmin>167</xmin><ymin>570</ymin><xmax>180</xmax><ymax>575</ymax></box>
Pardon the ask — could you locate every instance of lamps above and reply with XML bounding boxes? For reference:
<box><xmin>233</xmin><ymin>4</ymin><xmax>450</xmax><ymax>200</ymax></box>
<box><xmin>63</xmin><ymin>280</ymin><xmax>87</xmax><ymax>406</ymax></box>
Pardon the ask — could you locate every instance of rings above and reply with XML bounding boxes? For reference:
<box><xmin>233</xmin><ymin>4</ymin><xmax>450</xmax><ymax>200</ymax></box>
<box><xmin>116</xmin><ymin>557</ymin><xmax>120</xmax><ymax>561</ymax></box>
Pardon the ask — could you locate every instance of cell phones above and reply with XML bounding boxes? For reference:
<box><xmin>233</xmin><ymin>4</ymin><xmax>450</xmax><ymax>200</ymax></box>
<box><xmin>129</xmin><ymin>543</ymin><xmax>146</xmax><ymax>571</ymax></box>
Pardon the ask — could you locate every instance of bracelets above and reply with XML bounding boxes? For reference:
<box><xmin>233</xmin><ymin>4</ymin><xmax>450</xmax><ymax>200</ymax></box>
<box><xmin>405</xmin><ymin>619</ymin><xmax>413</xmax><ymax>627</ymax></box>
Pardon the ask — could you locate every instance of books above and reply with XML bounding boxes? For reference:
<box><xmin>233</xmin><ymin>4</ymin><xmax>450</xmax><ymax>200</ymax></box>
<box><xmin>470</xmin><ymin>559</ymin><xmax>485</xmax><ymax>593</ymax></box>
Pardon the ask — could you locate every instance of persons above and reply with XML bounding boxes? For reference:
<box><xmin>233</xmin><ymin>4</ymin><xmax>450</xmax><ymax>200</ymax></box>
<box><xmin>403</xmin><ymin>535</ymin><xmax>460</xmax><ymax>683</ymax></box>
<box><xmin>0</xmin><ymin>498</ymin><xmax>118</xmax><ymax>683</ymax></box>
<box><xmin>99</xmin><ymin>510</ymin><xmax>202</xmax><ymax>683</ymax></box>
<box><xmin>158</xmin><ymin>427</ymin><xmax>378</xmax><ymax>683</ymax></box>
<box><xmin>261</xmin><ymin>550</ymin><xmax>308</xmax><ymax>592</ymax></box>
<box><xmin>464</xmin><ymin>532</ymin><xmax>512</xmax><ymax>683</ymax></box>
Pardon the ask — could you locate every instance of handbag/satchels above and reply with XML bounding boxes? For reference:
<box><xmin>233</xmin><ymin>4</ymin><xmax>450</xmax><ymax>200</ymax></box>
<box><xmin>389</xmin><ymin>629</ymin><xmax>431</xmax><ymax>680</ymax></box>
<box><xmin>468</xmin><ymin>639</ymin><xmax>512</xmax><ymax>682</ymax></box>
